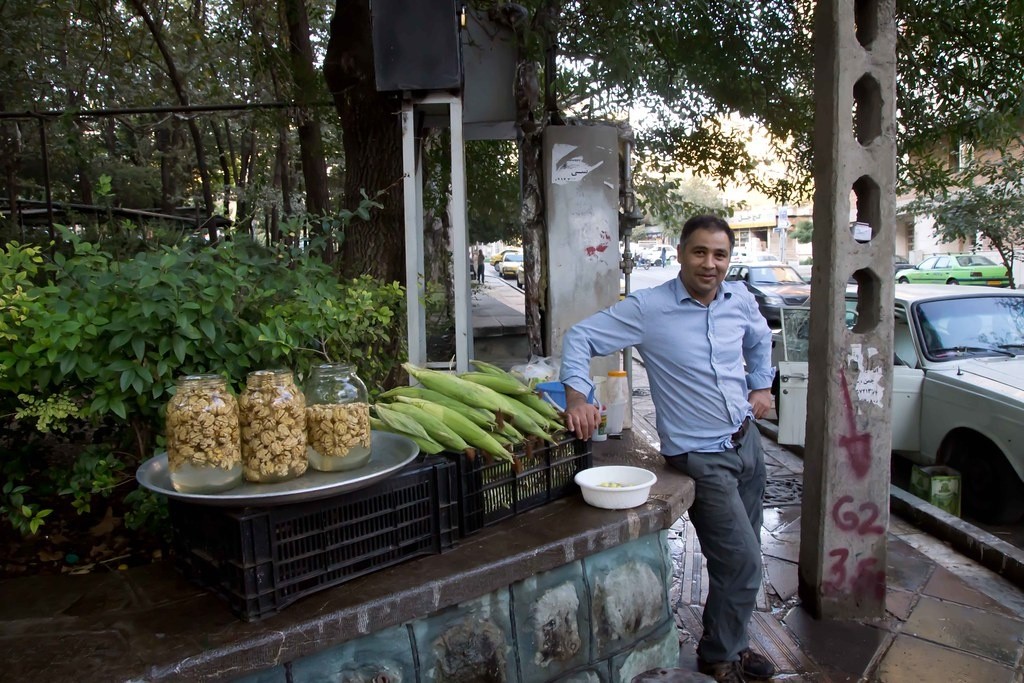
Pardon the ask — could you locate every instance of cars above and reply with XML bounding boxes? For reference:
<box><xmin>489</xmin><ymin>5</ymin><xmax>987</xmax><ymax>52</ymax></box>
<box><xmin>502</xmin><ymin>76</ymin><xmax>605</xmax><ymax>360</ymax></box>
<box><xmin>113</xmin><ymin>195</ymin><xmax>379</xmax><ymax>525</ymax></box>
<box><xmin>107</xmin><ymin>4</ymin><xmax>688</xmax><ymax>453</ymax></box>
<box><xmin>771</xmin><ymin>282</ymin><xmax>1024</xmax><ymax>527</ymax></box>
<box><xmin>619</xmin><ymin>240</ymin><xmax>678</xmax><ymax>268</ymax></box>
<box><xmin>724</xmin><ymin>263</ymin><xmax>810</xmax><ymax>329</ymax></box>
<box><xmin>478</xmin><ymin>243</ymin><xmax>525</xmax><ymax>289</ymax></box>
<box><xmin>730</xmin><ymin>246</ymin><xmax>780</xmax><ymax>264</ymax></box>
<box><xmin>895</xmin><ymin>251</ymin><xmax>1015</xmax><ymax>288</ymax></box>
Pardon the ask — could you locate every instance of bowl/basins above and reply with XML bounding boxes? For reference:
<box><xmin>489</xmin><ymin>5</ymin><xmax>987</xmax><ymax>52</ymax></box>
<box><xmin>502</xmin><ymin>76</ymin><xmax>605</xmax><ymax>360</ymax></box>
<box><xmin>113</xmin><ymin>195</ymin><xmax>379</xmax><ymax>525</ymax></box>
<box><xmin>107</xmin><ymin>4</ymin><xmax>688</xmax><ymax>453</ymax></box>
<box><xmin>574</xmin><ymin>465</ymin><xmax>657</xmax><ymax>509</ymax></box>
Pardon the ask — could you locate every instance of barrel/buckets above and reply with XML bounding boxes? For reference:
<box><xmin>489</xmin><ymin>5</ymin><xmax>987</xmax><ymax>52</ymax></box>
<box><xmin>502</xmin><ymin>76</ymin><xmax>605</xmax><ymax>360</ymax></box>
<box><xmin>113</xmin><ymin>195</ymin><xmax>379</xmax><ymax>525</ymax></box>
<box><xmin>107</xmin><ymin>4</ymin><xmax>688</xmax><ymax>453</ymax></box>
<box><xmin>536</xmin><ymin>381</ymin><xmax>602</xmax><ymax>415</ymax></box>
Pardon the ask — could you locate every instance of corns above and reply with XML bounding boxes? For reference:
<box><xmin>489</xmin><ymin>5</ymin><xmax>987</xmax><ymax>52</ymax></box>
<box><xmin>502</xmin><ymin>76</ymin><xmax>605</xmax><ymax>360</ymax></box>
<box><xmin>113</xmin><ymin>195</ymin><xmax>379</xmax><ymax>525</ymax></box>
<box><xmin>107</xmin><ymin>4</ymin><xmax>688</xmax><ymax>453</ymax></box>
<box><xmin>372</xmin><ymin>360</ymin><xmax>568</xmax><ymax>466</ymax></box>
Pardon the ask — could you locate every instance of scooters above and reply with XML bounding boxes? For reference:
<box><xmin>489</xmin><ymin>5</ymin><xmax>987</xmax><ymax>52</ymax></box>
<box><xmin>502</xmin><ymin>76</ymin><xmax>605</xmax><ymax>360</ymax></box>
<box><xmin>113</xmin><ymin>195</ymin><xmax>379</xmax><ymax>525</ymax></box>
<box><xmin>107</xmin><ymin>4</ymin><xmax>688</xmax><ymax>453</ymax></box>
<box><xmin>632</xmin><ymin>253</ymin><xmax>652</xmax><ymax>270</ymax></box>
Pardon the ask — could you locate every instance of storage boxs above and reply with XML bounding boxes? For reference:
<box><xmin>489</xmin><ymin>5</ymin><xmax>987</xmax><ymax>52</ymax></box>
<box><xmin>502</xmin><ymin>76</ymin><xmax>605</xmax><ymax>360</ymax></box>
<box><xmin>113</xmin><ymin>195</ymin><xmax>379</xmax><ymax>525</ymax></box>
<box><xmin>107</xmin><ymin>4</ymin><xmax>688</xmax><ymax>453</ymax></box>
<box><xmin>159</xmin><ymin>426</ymin><xmax>597</xmax><ymax>621</ymax></box>
<box><xmin>908</xmin><ymin>464</ymin><xmax>962</xmax><ymax>527</ymax></box>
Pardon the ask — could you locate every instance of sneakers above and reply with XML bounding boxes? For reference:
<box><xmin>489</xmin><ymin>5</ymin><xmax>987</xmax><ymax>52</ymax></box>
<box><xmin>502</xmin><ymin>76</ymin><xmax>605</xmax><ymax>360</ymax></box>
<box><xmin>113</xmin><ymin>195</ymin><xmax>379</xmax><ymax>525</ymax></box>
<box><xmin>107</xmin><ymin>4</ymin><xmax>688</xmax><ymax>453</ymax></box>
<box><xmin>739</xmin><ymin>647</ymin><xmax>776</xmax><ymax>678</ymax></box>
<box><xmin>696</xmin><ymin>655</ymin><xmax>746</xmax><ymax>683</ymax></box>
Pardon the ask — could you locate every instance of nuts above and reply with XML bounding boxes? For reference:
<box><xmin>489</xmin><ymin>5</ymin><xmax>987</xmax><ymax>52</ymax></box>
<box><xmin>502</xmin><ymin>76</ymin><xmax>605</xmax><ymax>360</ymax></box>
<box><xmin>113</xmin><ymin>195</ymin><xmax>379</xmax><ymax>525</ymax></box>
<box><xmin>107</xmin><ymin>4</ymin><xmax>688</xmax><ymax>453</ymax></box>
<box><xmin>165</xmin><ymin>378</ymin><xmax>241</xmax><ymax>474</ymax></box>
<box><xmin>307</xmin><ymin>403</ymin><xmax>372</xmax><ymax>458</ymax></box>
<box><xmin>239</xmin><ymin>370</ymin><xmax>309</xmax><ymax>481</ymax></box>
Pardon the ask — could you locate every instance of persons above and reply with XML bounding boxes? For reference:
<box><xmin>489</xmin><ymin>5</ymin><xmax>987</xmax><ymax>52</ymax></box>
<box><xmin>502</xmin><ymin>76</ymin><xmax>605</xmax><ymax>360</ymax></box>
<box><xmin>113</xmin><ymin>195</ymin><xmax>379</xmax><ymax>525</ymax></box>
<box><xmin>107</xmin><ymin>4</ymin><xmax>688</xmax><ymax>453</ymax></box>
<box><xmin>560</xmin><ymin>214</ymin><xmax>776</xmax><ymax>683</ymax></box>
<box><xmin>478</xmin><ymin>249</ymin><xmax>485</xmax><ymax>285</ymax></box>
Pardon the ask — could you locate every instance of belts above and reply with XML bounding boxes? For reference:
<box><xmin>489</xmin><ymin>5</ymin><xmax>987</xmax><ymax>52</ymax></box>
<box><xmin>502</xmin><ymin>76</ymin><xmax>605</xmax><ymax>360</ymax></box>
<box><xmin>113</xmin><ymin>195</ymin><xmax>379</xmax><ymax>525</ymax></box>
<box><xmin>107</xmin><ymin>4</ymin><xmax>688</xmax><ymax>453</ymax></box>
<box><xmin>732</xmin><ymin>417</ymin><xmax>750</xmax><ymax>440</ymax></box>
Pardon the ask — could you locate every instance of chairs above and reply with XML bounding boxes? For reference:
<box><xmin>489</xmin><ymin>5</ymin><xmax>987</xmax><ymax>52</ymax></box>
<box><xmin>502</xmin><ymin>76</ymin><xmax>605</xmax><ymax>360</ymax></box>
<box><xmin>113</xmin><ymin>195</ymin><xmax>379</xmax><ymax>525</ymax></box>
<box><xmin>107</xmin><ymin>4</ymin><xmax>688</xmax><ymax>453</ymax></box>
<box><xmin>893</xmin><ymin>323</ymin><xmax>921</xmax><ymax>368</ymax></box>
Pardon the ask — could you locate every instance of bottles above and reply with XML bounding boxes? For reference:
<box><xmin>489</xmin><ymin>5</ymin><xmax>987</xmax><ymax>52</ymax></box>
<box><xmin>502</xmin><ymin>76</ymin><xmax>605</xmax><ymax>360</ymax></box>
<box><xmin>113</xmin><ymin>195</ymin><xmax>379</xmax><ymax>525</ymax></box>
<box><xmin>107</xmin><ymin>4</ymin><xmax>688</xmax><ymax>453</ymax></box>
<box><xmin>304</xmin><ymin>360</ymin><xmax>372</xmax><ymax>471</ymax></box>
<box><xmin>240</xmin><ymin>367</ymin><xmax>309</xmax><ymax>484</ymax></box>
<box><xmin>592</xmin><ymin>371</ymin><xmax>632</xmax><ymax>441</ymax></box>
<box><xmin>166</xmin><ymin>374</ymin><xmax>242</xmax><ymax>494</ymax></box>
